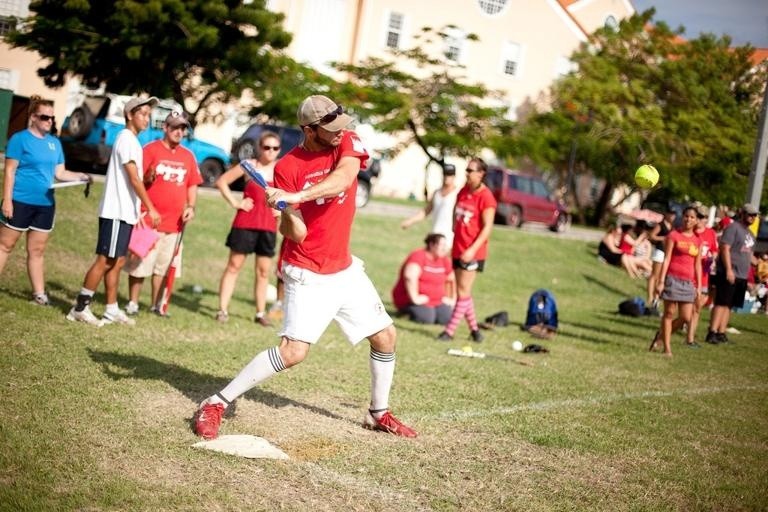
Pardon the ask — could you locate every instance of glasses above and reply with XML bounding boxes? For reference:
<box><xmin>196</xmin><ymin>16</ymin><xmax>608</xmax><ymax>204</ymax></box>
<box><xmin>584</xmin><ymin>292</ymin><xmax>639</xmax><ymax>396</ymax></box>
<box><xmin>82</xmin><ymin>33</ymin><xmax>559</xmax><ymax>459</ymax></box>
<box><xmin>32</xmin><ymin>114</ymin><xmax>55</xmax><ymax>121</ymax></box>
<box><xmin>465</xmin><ymin>168</ymin><xmax>476</xmax><ymax>173</ymax></box>
<box><xmin>171</xmin><ymin>110</ymin><xmax>189</xmax><ymax>120</ymax></box>
<box><xmin>262</xmin><ymin>145</ymin><xmax>280</xmax><ymax>151</ymax></box>
<box><xmin>309</xmin><ymin>106</ymin><xmax>343</xmax><ymax>126</ymax></box>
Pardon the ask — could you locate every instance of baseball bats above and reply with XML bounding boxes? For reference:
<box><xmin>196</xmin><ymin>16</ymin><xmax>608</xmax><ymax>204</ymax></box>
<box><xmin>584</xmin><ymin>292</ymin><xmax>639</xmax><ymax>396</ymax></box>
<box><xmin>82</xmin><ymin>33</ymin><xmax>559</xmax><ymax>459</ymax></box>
<box><xmin>156</xmin><ymin>220</ymin><xmax>186</xmax><ymax>315</ymax></box>
<box><xmin>240</xmin><ymin>158</ymin><xmax>287</xmax><ymax>210</ymax></box>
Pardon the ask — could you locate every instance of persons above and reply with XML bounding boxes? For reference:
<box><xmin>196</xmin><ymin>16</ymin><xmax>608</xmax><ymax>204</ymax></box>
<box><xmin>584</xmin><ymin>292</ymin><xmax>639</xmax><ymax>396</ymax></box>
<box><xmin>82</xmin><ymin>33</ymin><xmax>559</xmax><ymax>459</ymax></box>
<box><xmin>0</xmin><ymin>94</ymin><xmax>93</xmax><ymax>308</ymax></box>
<box><xmin>122</xmin><ymin>110</ymin><xmax>204</xmax><ymax>318</ymax></box>
<box><xmin>600</xmin><ymin>199</ymin><xmax>768</xmax><ymax>357</ymax></box>
<box><xmin>267</xmin><ymin>241</ymin><xmax>295</xmax><ymax>314</ymax></box>
<box><xmin>191</xmin><ymin>93</ymin><xmax>417</xmax><ymax>442</ymax></box>
<box><xmin>393</xmin><ymin>156</ymin><xmax>498</xmax><ymax>350</ymax></box>
<box><xmin>64</xmin><ymin>95</ymin><xmax>163</xmax><ymax>326</ymax></box>
<box><xmin>215</xmin><ymin>131</ymin><xmax>285</xmax><ymax>329</ymax></box>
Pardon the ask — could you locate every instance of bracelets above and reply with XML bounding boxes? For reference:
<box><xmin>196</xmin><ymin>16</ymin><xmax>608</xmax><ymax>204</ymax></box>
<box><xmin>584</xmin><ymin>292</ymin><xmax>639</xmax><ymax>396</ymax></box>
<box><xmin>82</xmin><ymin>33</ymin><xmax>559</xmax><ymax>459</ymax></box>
<box><xmin>187</xmin><ymin>205</ymin><xmax>196</xmax><ymax>211</ymax></box>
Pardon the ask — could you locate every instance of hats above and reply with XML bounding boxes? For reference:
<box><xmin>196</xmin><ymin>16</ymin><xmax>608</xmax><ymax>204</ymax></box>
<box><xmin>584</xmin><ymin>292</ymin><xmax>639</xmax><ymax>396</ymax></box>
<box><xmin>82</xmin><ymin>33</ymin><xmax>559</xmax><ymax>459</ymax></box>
<box><xmin>443</xmin><ymin>164</ymin><xmax>456</xmax><ymax>175</ymax></box>
<box><xmin>718</xmin><ymin>217</ymin><xmax>734</xmax><ymax>231</ymax></box>
<box><xmin>123</xmin><ymin>96</ymin><xmax>160</xmax><ymax>116</ymax></box>
<box><xmin>696</xmin><ymin>204</ymin><xmax>710</xmax><ymax>217</ymax></box>
<box><xmin>739</xmin><ymin>203</ymin><xmax>761</xmax><ymax>215</ymax></box>
<box><xmin>296</xmin><ymin>94</ymin><xmax>355</xmax><ymax>133</ymax></box>
<box><xmin>165</xmin><ymin>113</ymin><xmax>191</xmax><ymax>129</ymax></box>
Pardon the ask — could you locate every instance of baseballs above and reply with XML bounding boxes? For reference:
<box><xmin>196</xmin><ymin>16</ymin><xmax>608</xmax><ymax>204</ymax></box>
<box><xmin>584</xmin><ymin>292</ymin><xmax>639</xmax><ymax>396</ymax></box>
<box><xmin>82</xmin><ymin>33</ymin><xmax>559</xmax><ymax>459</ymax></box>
<box><xmin>463</xmin><ymin>346</ymin><xmax>472</xmax><ymax>355</ymax></box>
<box><xmin>635</xmin><ymin>164</ymin><xmax>660</xmax><ymax>189</ymax></box>
<box><xmin>512</xmin><ymin>341</ymin><xmax>523</xmax><ymax>351</ymax></box>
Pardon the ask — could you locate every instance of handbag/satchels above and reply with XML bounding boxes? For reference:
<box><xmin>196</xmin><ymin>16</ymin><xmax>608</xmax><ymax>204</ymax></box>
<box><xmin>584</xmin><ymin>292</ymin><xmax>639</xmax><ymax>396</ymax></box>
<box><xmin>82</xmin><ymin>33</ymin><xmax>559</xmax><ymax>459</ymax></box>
<box><xmin>618</xmin><ymin>296</ymin><xmax>644</xmax><ymax>317</ymax></box>
<box><xmin>526</xmin><ymin>289</ymin><xmax>558</xmax><ymax>328</ymax></box>
<box><xmin>128</xmin><ymin>226</ymin><xmax>160</xmax><ymax>260</ymax></box>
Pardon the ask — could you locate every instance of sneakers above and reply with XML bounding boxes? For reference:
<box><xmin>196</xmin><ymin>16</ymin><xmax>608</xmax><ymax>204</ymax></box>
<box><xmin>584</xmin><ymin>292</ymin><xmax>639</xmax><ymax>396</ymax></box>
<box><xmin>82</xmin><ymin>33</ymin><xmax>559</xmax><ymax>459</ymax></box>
<box><xmin>254</xmin><ymin>301</ymin><xmax>285</xmax><ymax>326</ymax></box>
<box><xmin>644</xmin><ymin>299</ymin><xmax>664</xmax><ymax>317</ymax></box>
<box><xmin>102</xmin><ymin>308</ymin><xmax>137</xmax><ymax>328</ymax></box>
<box><xmin>30</xmin><ymin>292</ymin><xmax>50</xmax><ymax>306</ymax></box>
<box><xmin>148</xmin><ymin>305</ymin><xmax>171</xmax><ymax>318</ymax></box>
<box><xmin>364</xmin><ymin>408</ymin><xmax>417</xmax><ymax>438</ymax></box>
<box><xmin>437</xmin><ymin>330</ymin><xmax>455</xmax><ymax>341</ymax></box>
<box><xmin>193</xmin><ymin>396</ymin><xmax>226</xmax><ymax>439</ymax></box>
<box><xmin>216</xmin><ymin>310</ymin><xmax>231</xmax><ymax>323</ymax></box>
<box><xmin>471</xmin><ymin>329</ymin><xmax>483</xmax><ymax>342</ymax></box>
<box><xmin>124</xmin><ymin>300</ymin><xmax>139</xmax><ymax>315</ymax></box>
<box><xmin>66</xmin><ymin>304</ymin><xmax>105</xmax><ymax>328</ymax></box>
<box><xmin>648</xmin><ymin>322</ymin><xmax>742</xmax><ymax>363</ymax></box>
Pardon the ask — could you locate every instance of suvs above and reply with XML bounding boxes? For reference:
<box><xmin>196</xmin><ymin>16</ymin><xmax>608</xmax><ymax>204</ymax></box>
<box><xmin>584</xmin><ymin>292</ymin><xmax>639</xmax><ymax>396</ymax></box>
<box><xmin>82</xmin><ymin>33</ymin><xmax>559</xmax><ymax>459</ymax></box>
<box><xmin>57</xmin><ymin>93</ymin><xmax>228</xmax><ymax>189</ymax></box>
<box><xmin>481</xmin><ymin>166</ymin><xmax>567</xmax><ymax>231</ymax></box>
<box><xmin>230</xmin><ymin>123</ymin><xmax>371</xmax><ymax>207</ymax></box>
